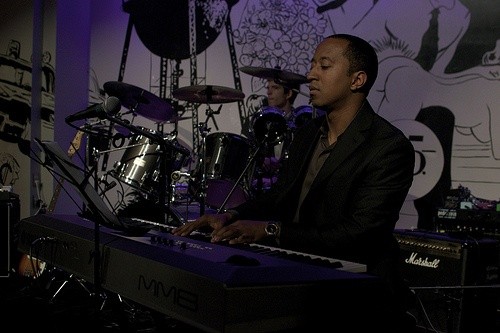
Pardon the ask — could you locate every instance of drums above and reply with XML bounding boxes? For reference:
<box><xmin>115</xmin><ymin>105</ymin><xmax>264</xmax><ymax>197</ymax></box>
<box><xmin>288</xmin><ymin>105</ymin><xmax>318</xmax><ymax>132</ymax></box>
<box><xmin>109</xmin><ymin>125</ymin><xmax>191</xmax><ymax>197</ymax></box>
<box><xmin>189</xmin><ymin>131</ymin><xmax>259</xmax><ymax>210</ymax></box>
<box><xmin>250</xmin><ymin>106</ymin><xmax>288</xmax><ymax>145</ymax></box>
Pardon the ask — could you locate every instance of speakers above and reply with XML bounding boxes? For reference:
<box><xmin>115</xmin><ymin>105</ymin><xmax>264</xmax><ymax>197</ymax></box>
<box><xmin>393</xmin><ymin>230</ymin><xmax>499</xmax><ymax>332</ymax></box>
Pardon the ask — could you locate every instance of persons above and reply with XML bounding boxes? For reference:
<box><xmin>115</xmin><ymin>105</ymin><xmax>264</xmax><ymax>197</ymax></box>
<box><xmin>171</xmin><ymin>34</ymin><xmax>415</xmax><ymax>306</ymax></box>
<box><xmin>263</xmin><ymin>77</ymin><xmax>300</xmax><ymax>127</ymax></box>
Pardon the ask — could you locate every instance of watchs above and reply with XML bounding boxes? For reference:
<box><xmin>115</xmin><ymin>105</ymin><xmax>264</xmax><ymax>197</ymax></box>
<box><xmin>264</xmin><ymin>220</ymin><xmax>279</xmax><ymax>239</ymax></box>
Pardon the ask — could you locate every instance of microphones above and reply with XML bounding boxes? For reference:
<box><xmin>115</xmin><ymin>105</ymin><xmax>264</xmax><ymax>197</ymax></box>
<box><xmin>65</xmin><ymin>96</ymin><xmax>121</xmax><ymax>122</ymax></box>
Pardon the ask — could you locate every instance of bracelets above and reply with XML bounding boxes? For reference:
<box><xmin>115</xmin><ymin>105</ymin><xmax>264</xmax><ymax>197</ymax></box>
<box><xmin>223</xmin><ymin>208</ymin><xmax>233</xmax><ymax>219</ymax></box>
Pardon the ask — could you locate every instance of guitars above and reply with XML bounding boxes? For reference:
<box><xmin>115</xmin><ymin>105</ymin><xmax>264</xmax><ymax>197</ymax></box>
<box><xmin>19</xmin><ymin>124</ymin><xmax>87</xmax><ymax>276</ymax></box>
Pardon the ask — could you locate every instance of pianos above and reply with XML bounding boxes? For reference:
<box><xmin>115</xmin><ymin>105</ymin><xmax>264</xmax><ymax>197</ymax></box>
<box><xmin>15</xmin><ymin>212</ymin><xmax>380</xmax><ymax>333</ymax></box>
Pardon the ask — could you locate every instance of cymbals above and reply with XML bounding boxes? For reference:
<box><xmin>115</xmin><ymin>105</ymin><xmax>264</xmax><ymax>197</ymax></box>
<box><xmin>101</xmin><ymin>81</ymin><xmax>177</xmax><ymax>123</ymax></box>
<box><xmin>238</xmin><ymin>65</ymin><xmax>309</xmax><ymax>83</ymax></box>
<box><xmin>172</xmin><ymin>84</ymin><xmax>246</xmax><ymax>105</ymax></box>
